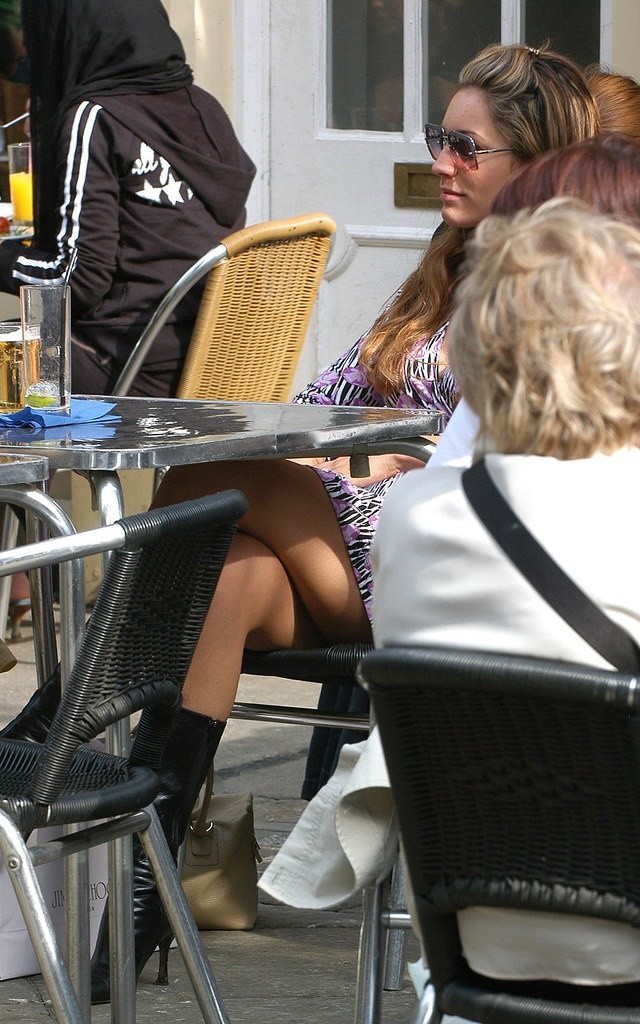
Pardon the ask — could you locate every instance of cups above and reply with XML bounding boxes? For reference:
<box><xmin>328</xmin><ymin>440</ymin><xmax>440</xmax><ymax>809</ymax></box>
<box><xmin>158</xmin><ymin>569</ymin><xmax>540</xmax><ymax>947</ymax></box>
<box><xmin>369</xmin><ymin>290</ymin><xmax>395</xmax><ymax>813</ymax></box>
<box><xmin>7</xmin><ymin>142</ymin><xmax>34</xmax><ymax>226</ymax></box>
<box><xmin>0</xmin><ymin>321</ymin><xmax>41</xmax><ymax>413</ymax></box>
<box><xmin>21</xmin><ymin>285</ymin><xmax>71</xmax><ymax>416</ymax></box>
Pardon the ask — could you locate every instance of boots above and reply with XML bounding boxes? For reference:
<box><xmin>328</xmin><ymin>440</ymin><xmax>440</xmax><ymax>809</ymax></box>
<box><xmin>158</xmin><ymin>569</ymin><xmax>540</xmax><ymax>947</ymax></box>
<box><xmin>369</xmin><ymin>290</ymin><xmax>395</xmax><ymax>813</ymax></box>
<box><xmin>84</xmin><ymin>699</ymin><xmax>228</xmax><ymax>1009</ymax></box>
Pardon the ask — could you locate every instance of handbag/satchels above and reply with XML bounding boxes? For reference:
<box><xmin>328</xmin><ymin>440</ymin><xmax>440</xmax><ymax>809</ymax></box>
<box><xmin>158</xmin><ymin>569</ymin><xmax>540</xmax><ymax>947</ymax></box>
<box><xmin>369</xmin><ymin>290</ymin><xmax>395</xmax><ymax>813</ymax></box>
<box><xmin>180</xmin><ymin>759</ymin><xmax>260</xmax><ymax>930</ymax></box>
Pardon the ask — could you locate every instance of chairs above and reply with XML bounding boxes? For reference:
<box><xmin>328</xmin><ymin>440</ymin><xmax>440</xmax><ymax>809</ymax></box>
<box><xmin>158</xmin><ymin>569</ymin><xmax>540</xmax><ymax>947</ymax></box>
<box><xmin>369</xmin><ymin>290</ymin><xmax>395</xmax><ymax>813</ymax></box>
<box><xmin>0</xmin><ymin>210</ymin><xmax>640</xmax><ymax>1024</ymax></box>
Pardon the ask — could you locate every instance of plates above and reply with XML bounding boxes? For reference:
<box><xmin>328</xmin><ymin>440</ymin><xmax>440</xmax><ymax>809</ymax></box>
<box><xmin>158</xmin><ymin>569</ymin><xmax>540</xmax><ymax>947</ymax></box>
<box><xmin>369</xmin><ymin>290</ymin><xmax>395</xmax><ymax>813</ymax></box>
<box><xmin>0</xmin><ymin>226</ymin><xmax>34</xmax><ymax>240</ymax></box>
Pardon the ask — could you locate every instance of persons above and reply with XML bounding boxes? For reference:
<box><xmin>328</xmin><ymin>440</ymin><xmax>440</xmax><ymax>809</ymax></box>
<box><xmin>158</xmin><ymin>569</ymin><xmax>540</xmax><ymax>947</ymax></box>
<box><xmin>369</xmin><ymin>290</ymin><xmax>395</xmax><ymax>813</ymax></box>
<box><xmin>1</xmin><ymin>49</ymin><xmax>640</xmax><ymax>1002</ymax></box>
<box><xmin>0</xmin><ymin>1</ymin><xmax>257</xmax><ymax>620</ymax></box>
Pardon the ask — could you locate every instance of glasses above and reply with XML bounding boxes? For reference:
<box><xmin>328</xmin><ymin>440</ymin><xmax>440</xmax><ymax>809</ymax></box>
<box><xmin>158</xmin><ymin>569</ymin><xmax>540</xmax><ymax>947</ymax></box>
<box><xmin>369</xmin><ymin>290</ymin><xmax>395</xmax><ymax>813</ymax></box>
<box><xmin>423</xmin><ymin>122</ymin><xmax>524</xmax><ymax>173</ymax></box>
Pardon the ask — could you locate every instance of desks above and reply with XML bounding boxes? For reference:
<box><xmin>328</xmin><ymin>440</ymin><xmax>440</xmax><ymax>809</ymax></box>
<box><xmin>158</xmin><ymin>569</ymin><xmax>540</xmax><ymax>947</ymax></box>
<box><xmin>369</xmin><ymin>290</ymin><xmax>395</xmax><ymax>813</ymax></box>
<box><xmin>0</xmin><ymin>392</ymin><xmax>443</xmax><ymax>1024</ymax></box>
